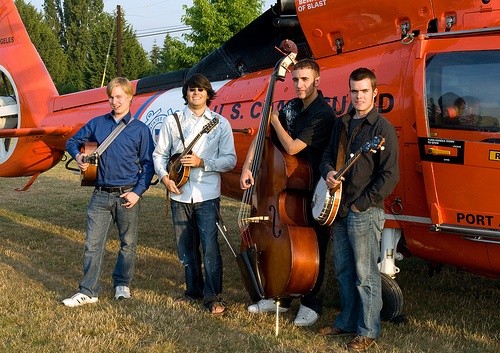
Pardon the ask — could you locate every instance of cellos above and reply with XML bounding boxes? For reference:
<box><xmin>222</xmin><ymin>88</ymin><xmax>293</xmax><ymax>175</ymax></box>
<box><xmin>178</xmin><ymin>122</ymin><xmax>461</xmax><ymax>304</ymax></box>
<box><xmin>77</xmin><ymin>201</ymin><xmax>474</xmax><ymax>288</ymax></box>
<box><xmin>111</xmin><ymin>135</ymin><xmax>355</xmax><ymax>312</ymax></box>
<box><xmin>237</xmin><ymin>39</ymin><xmax>320</xmax><ymax>338</ymax></box>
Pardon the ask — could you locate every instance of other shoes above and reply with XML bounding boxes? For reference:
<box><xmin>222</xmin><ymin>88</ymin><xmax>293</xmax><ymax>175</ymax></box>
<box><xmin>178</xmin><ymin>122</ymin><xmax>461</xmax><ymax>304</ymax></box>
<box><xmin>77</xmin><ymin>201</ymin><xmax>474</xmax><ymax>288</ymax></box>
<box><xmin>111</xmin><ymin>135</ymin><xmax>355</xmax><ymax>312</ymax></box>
<box><xmin>176</xmin><ymin>293</ymin><xmax>193</xmax><ymax>301</ymax></box>
<box><xmin>204</xmin><ymin>294</ymin><xmax>226</xmax><ymax>314</ymax></box>
<box><xmin>315</xmin><ymin>323</ymin><xmax>358</xmax><ymax>336</ymax></box>
<box><xmin>346</xmin><ymin>335</ymin><xmax>382</xmax><ymax>350</ymax></box>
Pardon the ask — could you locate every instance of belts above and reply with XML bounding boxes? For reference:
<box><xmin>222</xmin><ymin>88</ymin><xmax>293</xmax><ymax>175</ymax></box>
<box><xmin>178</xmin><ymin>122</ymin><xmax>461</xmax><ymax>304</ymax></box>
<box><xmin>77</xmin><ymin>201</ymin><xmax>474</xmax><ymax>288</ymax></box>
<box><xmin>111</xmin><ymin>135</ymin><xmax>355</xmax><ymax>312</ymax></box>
<box><xmin>94</xmin><ymin>185</ymin><xmax>134</xmax><ymax>192</ymax></box>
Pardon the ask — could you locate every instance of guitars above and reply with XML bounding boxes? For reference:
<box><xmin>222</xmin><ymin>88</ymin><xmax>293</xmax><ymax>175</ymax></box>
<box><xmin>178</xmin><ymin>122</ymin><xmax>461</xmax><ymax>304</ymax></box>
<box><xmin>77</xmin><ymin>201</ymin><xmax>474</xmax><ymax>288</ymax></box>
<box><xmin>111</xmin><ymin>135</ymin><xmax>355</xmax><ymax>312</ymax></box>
<box><xmin>80</xmin><ymin>141</ymin><xmax>99</xmax><ymax>187</ymax></box>
<box><xmin>166</xmin><ymin>116</ymin><xmax>220</xmax><ymax>190</ymax></box>
<box><xmin>311</xmin><ymin>134</ymin><xmax>385</xmax><ymax>226</ymax></box>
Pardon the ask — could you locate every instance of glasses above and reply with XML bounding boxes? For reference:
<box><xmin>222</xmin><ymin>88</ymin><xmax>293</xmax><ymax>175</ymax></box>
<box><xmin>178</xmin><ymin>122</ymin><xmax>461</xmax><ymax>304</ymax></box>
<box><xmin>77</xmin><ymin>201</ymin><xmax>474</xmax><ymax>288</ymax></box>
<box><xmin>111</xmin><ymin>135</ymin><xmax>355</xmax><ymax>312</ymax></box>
<box><xmin>190</xmin><ymin>88</ymin><xmax>203</xmax><ymax>92</ymax></box>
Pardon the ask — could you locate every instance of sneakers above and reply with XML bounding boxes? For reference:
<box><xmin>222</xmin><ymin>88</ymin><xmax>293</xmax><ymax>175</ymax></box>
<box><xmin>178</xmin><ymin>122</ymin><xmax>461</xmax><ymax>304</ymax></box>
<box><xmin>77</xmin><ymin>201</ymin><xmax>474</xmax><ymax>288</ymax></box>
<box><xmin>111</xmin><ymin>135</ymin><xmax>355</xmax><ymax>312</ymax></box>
<box><xmin>60</xmin><ymin>292</ymin><xmax>100</xmax><ymax>307</ymax></box>
<box><xmin>294</xmin><ymin>304</ymin><xmax>321</xmax><ymax>327</ymax></box>
<box><xmin>114</xmin><ymin>286</ymin><xmax>132</xmax><ymax>301</ymax></box>
<box><xmin>248</xmin><ymin>298</ymin><xmax>288</xmax><ymax>313</ymax></box>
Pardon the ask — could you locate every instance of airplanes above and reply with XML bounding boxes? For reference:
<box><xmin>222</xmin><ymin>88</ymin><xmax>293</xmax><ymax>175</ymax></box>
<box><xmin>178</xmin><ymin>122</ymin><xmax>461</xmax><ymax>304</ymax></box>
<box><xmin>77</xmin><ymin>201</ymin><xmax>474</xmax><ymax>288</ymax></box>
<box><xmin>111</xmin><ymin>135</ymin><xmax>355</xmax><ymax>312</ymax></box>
<box><xmin>0</xmin><ymin>0</ymin><xmax>500</xmax><ymax>321</ymax></box>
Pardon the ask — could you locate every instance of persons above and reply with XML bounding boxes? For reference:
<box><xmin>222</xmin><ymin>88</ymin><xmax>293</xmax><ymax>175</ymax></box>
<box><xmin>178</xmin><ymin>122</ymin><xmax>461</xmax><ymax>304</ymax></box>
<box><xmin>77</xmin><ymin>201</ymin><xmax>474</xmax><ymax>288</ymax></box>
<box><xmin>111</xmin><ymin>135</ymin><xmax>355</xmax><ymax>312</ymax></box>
<box><xmin>433</xmin><ymin>92</ymin><xmax>465</xmax><ymax>127</ymax></box>
<box><xmin>320</xmin><ymin>67</ymin><xmax>398</xmax><ymax>351</ymax></box>
<box><xmin>61</xmin><ymin>77</ymin><xmax>154</xmax><ymax>307</ymax></box>
<box><xmin>239</xmin><ymin>59</ymin><xmax>336</xmax><ymax>327</ymax></box>
<box><xmin>152</xmin><ymin>75</ymin><xmax>236</xmax><ymax>313</ymax></box>
